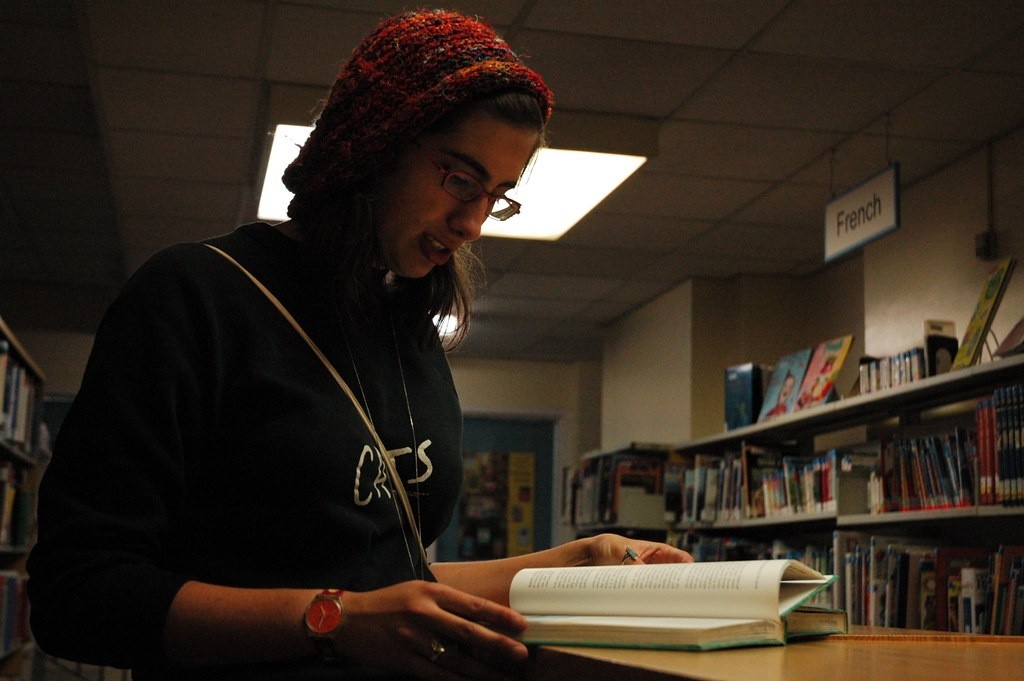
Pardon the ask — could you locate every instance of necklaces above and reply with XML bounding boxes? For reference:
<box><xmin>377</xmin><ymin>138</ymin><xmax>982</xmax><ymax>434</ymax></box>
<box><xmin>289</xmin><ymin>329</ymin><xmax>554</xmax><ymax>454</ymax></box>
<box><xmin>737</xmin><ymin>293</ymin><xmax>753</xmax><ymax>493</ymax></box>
<box><xmin>339</xmin><ymin>305</ymin><xmax>425</xmax><ymax>580</ymax></box>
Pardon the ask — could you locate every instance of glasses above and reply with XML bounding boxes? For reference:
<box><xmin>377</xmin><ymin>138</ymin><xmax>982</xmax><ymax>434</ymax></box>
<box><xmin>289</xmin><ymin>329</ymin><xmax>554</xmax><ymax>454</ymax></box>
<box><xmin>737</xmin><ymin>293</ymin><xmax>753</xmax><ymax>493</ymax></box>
<box><xmin>413</xmin><ymin>139</ymin><xmax>520</xmax><ymax>222</ymax></box>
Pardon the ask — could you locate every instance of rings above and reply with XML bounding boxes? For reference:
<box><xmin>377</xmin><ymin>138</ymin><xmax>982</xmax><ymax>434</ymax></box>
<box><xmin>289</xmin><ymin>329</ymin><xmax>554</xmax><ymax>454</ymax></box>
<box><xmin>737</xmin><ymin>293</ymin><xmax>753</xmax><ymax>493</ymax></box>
<box><xmin>621</xmin><ymin>546</ymin><xmax>638</xmax><ymax>565</ymax></box>
<box><xmin>429</xmin><ymin>640</ymin><xmax>446</xmax><ymax>662</ymax></box>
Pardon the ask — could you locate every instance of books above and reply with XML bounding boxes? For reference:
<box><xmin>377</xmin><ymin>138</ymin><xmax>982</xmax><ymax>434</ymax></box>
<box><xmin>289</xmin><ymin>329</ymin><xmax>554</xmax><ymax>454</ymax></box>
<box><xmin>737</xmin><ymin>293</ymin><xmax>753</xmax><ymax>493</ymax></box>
<box><xmin>860</xmin><ymin>319</ymin><xmax>955</xmax><ymax>394</ymax></box>
<box><xmin>950</xmin><ymin>258</ymin><xmax>1016</xmax><ymax>371</ymax></box>
<box><xmin>683</xmin><ymin>385</ymin><xmax>1024</xmax><ymax>635</ymax></box>
<box><xmin>506</xmin><ymin>560</ymin><xmax>849</xmax><ymax>651</ymax></box>
<box><xmin>559</xmin><ymin>439</ymin><xmax>684</xmax><ymax>550</ymax></box>
<box><xmin>723</xmin><ymin>335</ymin><xmax>856</xmax><ymax>429</ymax></box>
<box><xmin>0</xmin><ymin>353</ymin><xmax>42</xmax><ymax>656</ymax></box>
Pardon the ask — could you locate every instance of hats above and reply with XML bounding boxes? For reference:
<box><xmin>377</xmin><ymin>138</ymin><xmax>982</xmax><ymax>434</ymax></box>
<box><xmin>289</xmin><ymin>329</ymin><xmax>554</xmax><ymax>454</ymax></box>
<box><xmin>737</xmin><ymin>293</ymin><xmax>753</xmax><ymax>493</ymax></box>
<box><xmin>282</xmin><ymin>7</ymin><xmax>554</xmax><ymax>201</ymax></box>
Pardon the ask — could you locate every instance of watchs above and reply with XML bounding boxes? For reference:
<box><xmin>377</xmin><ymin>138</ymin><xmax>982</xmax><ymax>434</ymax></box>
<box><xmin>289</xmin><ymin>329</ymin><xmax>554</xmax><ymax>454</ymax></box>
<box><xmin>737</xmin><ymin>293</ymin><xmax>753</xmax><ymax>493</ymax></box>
<box><xmin>304</xmin><ymin>588</ymin><xmax>347</xmax><ymax>663</ymax></box>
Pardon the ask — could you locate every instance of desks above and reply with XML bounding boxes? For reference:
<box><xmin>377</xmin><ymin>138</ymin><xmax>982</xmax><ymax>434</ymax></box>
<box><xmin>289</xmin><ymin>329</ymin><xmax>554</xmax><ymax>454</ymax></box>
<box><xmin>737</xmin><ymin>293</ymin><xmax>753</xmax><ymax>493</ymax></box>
<box><xmin>539</xmin><ymin>626</ymin><xmax>1023</xmax><ymax>681</ymax></box>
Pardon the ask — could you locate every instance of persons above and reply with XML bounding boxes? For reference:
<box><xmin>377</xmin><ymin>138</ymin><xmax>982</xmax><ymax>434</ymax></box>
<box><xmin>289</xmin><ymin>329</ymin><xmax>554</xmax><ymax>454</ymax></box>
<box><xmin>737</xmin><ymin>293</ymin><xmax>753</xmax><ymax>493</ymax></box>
<box><xmin>765</xmin><ymin>374</ymin><xmax>795</xmax><ymax>417</ymax></box>
<box><xmin>26</xmin><ymin>9</ymin><xmax>695</xmax><ymax>681</ymax></box>
<box><xmin>808</xmin><ymin>355</ymin><xmax>837</xmax><ymax>400</ymax></box>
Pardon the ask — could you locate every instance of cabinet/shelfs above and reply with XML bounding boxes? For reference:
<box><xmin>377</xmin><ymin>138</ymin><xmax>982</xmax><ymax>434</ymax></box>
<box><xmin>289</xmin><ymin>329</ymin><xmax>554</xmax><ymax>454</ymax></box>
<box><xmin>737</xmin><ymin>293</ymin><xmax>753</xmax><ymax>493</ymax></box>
<box><xmin>560</xmin><ymin>440</ymin><xmax>672</xmax><ymax>534</ymax></box>
<box><xmin>1</xmin><ymin>318</ymin><xmax>45</xmax><ymax>681</ymax></box>
<box><xmin>673</xmin><ymin>354</ymin><xmax>1024</xmax><ymax>529</ymax></box>
